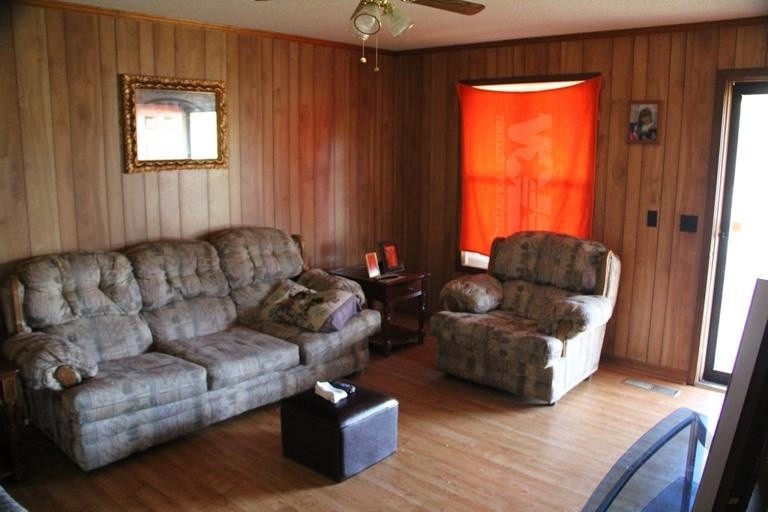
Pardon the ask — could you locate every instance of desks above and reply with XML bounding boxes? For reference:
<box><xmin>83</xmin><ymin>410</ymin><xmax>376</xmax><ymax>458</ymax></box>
<box><xmin>327</xmin><ymin>261</ymin><xmax>432</xmax><ymax>355</ymax></box>
<box><xmin>580</xmin><ymin>405</ymin><xmax>767</xmax><ymax>512</ymax></box>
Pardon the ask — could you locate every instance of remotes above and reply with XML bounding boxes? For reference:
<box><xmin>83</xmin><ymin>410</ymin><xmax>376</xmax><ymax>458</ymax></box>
<box><xmin>329</xmin><ymin>378</ymin><xmax>356</xmax><ymax>394</ymax></box>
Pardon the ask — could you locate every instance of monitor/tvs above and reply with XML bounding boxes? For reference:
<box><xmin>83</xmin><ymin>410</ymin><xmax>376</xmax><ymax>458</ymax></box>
<box><xmin>691</xmin><ymin>280</ymin><xmax>768</xmax><ymax>512</ymax></box>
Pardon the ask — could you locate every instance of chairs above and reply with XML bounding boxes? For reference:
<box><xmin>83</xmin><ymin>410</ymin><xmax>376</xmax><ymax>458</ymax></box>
<box><xmin>429</xmin><ymin>230</ymin><xmax>624</xmax><ymax>407</ymax></box>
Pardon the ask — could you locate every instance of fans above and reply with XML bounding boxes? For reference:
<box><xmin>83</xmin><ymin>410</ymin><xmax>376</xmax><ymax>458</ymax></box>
<box><xmin>339</xmin><ymin>0</ymin><xmax>489</xmax><ymax>22</ymax></box>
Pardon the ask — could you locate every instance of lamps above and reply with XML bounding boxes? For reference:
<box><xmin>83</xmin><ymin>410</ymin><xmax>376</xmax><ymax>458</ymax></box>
<box><xmin>348</xmin><ymin>1</ymin><xmax>415</xmax><ymax>72</ymax></box>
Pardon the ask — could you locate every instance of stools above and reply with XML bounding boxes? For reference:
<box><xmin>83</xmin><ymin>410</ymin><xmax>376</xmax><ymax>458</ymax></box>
<box><xmin>278</xmin><ymin>378</ymin><xmax>402</xmax><ymax>483</ymax></box>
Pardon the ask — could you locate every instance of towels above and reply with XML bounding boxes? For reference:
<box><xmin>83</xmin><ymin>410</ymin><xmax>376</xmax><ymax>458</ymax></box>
<box><xmin>314</xmin><ymin>379</ymin><xmax>347</xmax><ymax>404</ymax></box>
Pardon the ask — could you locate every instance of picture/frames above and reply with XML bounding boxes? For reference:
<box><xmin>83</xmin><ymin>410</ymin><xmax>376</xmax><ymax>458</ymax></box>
<box><xmin>364</xmin><ymin>250</ymin><xmax>381</xmax><ymax>279</ymax></box>
<box><xmin>625</xmin><ymin>99</ymin><xmax>664</xmax><ymax>144</ymax></box>
<box><xmin>380</xmin><ymin>239</ymin><xmax>405</xmax><ymax>275</ymax></box>
<box><xmin>120</xmin><ymin>71</ymin><xmax>231</xmax><ymax>174</ymax></box>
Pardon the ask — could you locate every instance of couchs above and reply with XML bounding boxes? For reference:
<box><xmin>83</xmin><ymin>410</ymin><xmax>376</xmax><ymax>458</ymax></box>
<box><xmin>0</xmin><ymin>220</ymin><xmax>385</xmax><ymax>477</ymax></box>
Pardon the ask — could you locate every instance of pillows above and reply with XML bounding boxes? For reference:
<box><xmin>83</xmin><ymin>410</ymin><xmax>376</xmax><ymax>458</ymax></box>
<box><xmin>256</xmin><ymin>277</ymin><xmax>363</xmax><ymax>333</ymax></box>
<box><xmin>297</xmin><ymin>266</ymin><xmax>367</xmax><ymax>311</ymax></box>
